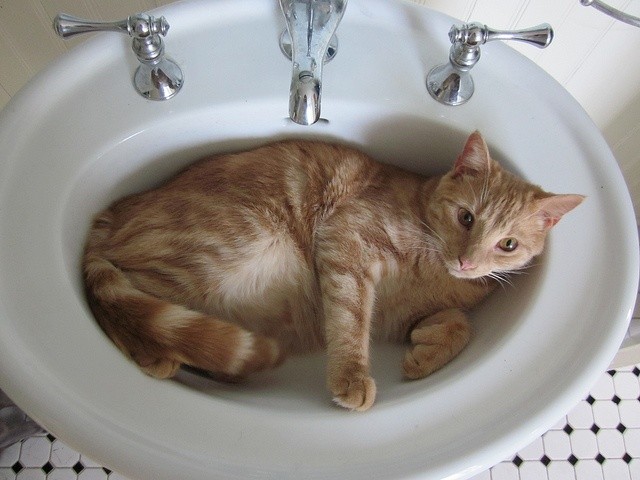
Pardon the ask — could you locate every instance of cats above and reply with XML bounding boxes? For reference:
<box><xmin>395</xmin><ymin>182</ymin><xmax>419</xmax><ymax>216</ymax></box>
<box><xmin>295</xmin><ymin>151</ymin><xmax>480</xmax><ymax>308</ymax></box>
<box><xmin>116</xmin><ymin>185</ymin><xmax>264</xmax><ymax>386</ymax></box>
<box><xmin>83</xmin><ymin>128</ymin><xmax>589</xmax><ymax>413</ymax></box>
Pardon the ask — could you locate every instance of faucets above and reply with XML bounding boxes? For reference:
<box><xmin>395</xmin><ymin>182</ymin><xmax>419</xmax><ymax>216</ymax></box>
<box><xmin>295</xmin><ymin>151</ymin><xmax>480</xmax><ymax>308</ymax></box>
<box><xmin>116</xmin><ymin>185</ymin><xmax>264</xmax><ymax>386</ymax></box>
<box><xmin>278</xmin><ymin>0</ymin><xmax>348</xmax><ymax>126</ymax></box>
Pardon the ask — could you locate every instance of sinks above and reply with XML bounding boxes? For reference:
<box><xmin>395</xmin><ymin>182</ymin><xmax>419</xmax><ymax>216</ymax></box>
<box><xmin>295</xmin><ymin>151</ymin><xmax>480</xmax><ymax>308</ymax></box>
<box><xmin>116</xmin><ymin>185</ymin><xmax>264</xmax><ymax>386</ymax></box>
<box><xmin>0</xmin><ymin>0</ymin><xmax>640</xmax><ymax>480</ymax></box>
